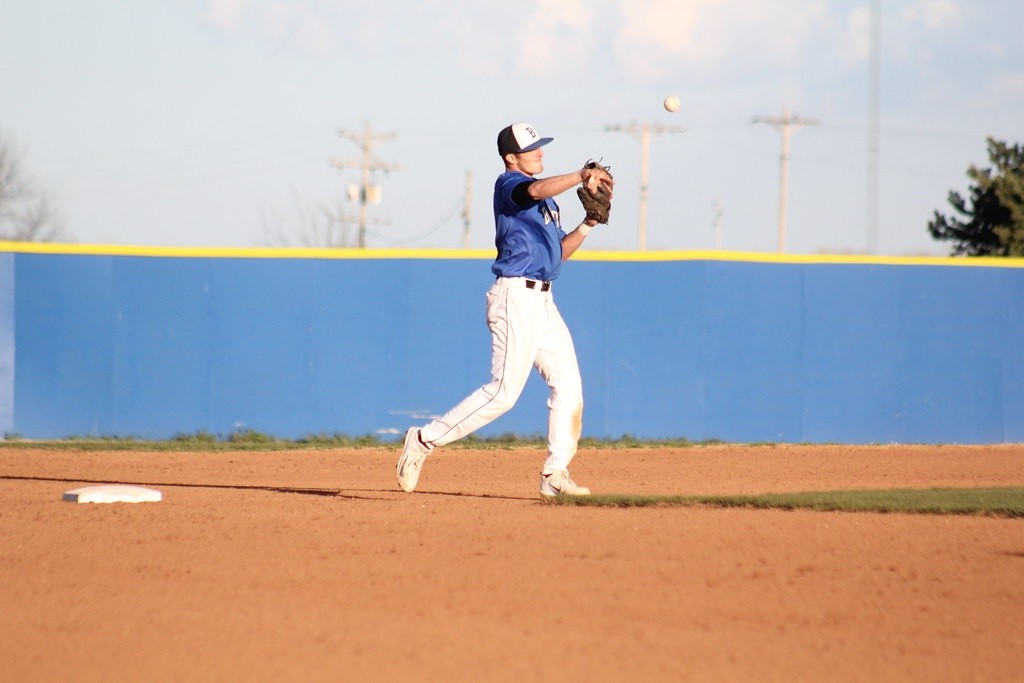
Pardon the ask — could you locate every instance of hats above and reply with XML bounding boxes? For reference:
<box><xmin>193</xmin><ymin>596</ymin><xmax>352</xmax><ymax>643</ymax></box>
<box><xmin>497</xmin><ymin>124</ymin><xmax>554</xmax><ymax>156</ymax></box>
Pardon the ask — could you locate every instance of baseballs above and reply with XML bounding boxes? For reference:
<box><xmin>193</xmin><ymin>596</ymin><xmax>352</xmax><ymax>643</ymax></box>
<box><xmin>664</xmin><ymin>96</ymin><xmax>681</xmax><ymax>112</ymax></box>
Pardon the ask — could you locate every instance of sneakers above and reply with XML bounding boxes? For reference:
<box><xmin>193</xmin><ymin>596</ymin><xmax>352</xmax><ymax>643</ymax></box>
<box><xmin>396</xmin><ymin>427</ymin><xmax>432</xmax><ymax>492</ymax></box>
<box><xmin>540</xmin><ymin>469</ymin><xmax>591</xmax><ymax>498</ymax></box>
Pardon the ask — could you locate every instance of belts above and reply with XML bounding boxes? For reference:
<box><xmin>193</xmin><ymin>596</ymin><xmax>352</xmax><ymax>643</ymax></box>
<box><xmin>496</xmin><ymin>276</ymin><xmax>550</xmax><ymax>293</ymax></box>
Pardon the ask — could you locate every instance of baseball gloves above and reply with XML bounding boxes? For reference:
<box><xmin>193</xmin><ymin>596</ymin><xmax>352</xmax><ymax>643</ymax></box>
<box><xmin>576</xmin><ymin>162</ymin><xmax>614</xmax><ymax>224</ymax></box>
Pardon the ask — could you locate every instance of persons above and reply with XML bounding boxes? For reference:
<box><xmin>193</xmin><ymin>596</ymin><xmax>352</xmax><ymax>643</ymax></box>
<box><xmin>396</xmin><ymin>122</ymin><xmax>616</xmax><ymax>499</ymax></box>
